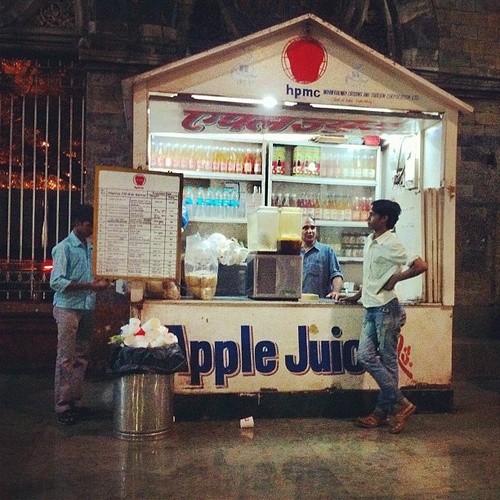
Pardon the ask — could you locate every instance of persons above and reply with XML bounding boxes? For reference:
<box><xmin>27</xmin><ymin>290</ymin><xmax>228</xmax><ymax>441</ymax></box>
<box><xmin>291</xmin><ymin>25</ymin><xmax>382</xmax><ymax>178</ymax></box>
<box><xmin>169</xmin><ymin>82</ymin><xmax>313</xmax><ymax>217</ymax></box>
<box><xmin>50</xmin><ymin>205</ymin><xmax>118</xmax><ymax>425</ymax></box>
<box><xmin>336</xmin><ymin>200</ymin><xmax>427</xmax><ymax>434</ymax></box>
<box><xmin>300</xmin><ymin>216</ymin><xmax>343</xmax><ymax>300</ymax></box>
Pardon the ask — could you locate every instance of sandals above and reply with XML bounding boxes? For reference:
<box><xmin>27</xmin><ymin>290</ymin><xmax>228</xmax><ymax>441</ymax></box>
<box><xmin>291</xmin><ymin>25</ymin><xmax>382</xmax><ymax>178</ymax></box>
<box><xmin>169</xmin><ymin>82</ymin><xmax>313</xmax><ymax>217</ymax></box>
<box><xmin>389</xmin><ymin>402</ymin><xmax>416</xmax><ymax>433</ymax></box>
<box><xmin>361</xmin><ymin>412</ymin><xmax>389</xmax><ymax>427</ymax></box>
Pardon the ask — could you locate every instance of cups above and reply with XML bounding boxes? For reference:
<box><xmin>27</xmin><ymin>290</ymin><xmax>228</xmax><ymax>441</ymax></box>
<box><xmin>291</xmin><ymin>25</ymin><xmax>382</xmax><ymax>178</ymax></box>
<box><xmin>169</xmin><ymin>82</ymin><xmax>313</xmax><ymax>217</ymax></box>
<box><xmin>120</xmin><ymin>318</ymin><xmax>178</xmax><ymax>348</ymax></box>
<box><xmin>240</xmin><ymin>416</ymin><xmax>254</xmax><ymax>428</ymax></box>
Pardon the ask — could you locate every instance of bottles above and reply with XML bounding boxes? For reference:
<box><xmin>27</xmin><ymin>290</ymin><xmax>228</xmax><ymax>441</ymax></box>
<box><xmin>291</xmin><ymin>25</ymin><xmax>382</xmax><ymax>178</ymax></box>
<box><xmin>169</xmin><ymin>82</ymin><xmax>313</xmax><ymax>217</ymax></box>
<box><xmin>182</xmin><ymin>184</ymin><xmax>261</xmax><ymax>221</ymax></box>
<box><xmin>319</xmin><ymin>150</ymin><xmax>376</xmax><ymax>180</ymax></box>
<box><xmin>150</xmin><ymin>140</ymin><xmax>261</xmax><ymax>174</ymax></box>
<box><xmin>341</xmin><ymin>231</ymin><xmax>373</xmax><ymax>258</ymax></box>
<box><xmin>272</xmin><ymin>192</ymin><xmax>374</xmax><ymax>222</ymax></box>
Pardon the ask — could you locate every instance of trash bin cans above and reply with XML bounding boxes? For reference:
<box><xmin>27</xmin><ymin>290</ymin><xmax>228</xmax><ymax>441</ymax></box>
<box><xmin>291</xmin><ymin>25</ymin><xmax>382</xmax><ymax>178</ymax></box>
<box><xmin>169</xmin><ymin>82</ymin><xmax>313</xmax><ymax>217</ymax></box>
<box><xmin>108</xmin><ymin>341</ymin><xmax>186</xmax><ymax>442</ymax></box>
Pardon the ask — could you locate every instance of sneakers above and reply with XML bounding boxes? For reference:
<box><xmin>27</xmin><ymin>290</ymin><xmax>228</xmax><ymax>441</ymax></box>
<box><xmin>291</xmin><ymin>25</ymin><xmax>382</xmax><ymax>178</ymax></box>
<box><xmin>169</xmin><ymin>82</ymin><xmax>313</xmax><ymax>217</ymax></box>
<box><xmin>56</xmin><ymin>410</ymin><xmax>75</xmax><ymax>426</ymax></box>
<box><xmin>71</xmin><ymin>406</ymin><xmax>93</xmax><ymax>419</ymax></box>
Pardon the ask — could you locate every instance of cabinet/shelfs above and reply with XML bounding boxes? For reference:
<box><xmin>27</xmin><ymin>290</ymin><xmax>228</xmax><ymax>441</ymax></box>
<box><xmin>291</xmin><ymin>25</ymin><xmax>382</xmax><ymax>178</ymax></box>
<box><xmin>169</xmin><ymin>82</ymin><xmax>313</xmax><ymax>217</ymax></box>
<box><xmin>149</xmin><ymin>131</ymin><xmax>267</xmax><ymax>258</ymax></box>
<box><xmin>268</xmin><ymin>141</ymin><xmax>381</xmax><ymax>263</ymax></box>
